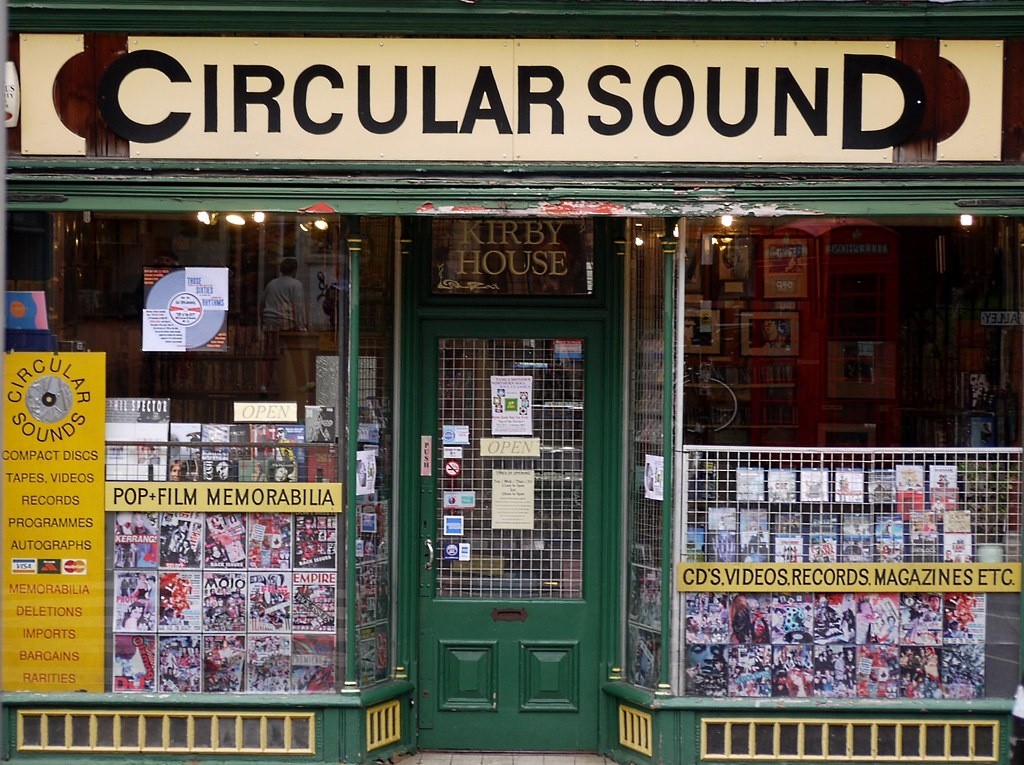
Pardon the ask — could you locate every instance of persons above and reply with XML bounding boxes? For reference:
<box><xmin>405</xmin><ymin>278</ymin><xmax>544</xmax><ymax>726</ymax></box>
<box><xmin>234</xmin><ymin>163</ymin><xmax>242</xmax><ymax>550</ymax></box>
<box><xmin>259</xmin><ymin>254</ymin><xmax>309</xmax><ymax>397</ymax></box>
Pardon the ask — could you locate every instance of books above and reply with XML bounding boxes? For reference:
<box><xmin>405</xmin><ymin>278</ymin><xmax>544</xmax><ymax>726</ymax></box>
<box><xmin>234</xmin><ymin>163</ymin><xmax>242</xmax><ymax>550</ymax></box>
<box><xmin>106</xmin><ymin>397</ymin><xmax>393</xmax><ymax>695</ymax></box>
<box><xmin>624</xmin><ymin>453</ymin><xmax>985</xmax><ymax>699</ymax></box>
